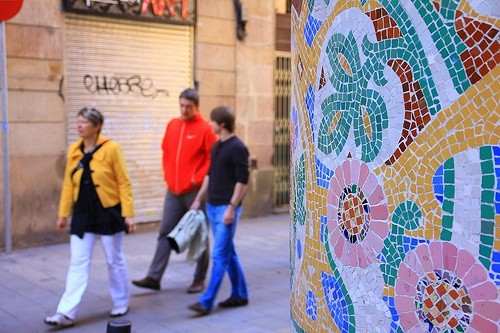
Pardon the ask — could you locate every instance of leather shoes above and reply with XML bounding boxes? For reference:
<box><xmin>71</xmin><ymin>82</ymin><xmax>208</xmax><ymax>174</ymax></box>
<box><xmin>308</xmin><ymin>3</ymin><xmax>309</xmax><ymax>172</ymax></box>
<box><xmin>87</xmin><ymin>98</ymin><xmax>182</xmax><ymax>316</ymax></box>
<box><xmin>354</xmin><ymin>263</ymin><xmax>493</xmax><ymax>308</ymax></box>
<box><xmin>219</xmin><ymin>296</ymin><xmax>249</xmax><ymax>307</ymax></box>
<box><xmin>187</xmin><ymin>300</ymin><xmax>211</xmax><ymax>315</ymax></box>
<box><xmin>187</xmin><ymin>280</ymin><xmax>204</xmax><ymax>293</ymax></box>
<box><xmin>132</xmin><ymin>276</ymin><xmax>160</xmax><ymax>290</ymax></box>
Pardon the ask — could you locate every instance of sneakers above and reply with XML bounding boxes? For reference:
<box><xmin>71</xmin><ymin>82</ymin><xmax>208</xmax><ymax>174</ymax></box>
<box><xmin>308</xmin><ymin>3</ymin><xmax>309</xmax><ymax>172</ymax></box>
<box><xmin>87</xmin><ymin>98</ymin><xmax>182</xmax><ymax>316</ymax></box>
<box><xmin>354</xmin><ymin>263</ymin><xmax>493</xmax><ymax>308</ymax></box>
<box><xmin>110</xmin><ymin>307</ymin><xmax>129</xmax><ymax>315</ymax></box>
<box><xmin>44</xmin><ymin>314</ymin><xmax>73</xmax><ymax>325</ymax></box>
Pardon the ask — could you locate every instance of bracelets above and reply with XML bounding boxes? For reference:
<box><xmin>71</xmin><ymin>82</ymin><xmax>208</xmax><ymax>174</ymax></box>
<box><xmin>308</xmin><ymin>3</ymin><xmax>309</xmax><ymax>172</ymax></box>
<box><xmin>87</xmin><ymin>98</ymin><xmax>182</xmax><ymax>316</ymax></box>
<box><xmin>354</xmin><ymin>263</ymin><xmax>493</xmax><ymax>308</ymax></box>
<box><xmin>229</xmin><ymin>201</ymin><xmax>236</xmax><ymax>210</ymax></box>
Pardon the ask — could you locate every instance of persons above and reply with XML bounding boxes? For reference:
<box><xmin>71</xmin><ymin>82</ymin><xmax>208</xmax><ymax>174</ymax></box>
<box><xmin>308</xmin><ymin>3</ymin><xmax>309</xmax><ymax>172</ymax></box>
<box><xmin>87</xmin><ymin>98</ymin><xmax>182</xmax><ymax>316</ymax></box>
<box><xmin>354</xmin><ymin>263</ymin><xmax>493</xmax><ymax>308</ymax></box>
<box><xmin>128</xmin><ymin>87</ymin><xmax>219</xmax><ymax>293</ymax></box>
<box><xmin>42</xmin><ymin>104</ymin><xmax>137</xmax><ymax>326</ymax></box>
<box><xmin>180</xmin><ymin>102</ymin><xmax>254</xmax><ymax>314</ymax></box>
